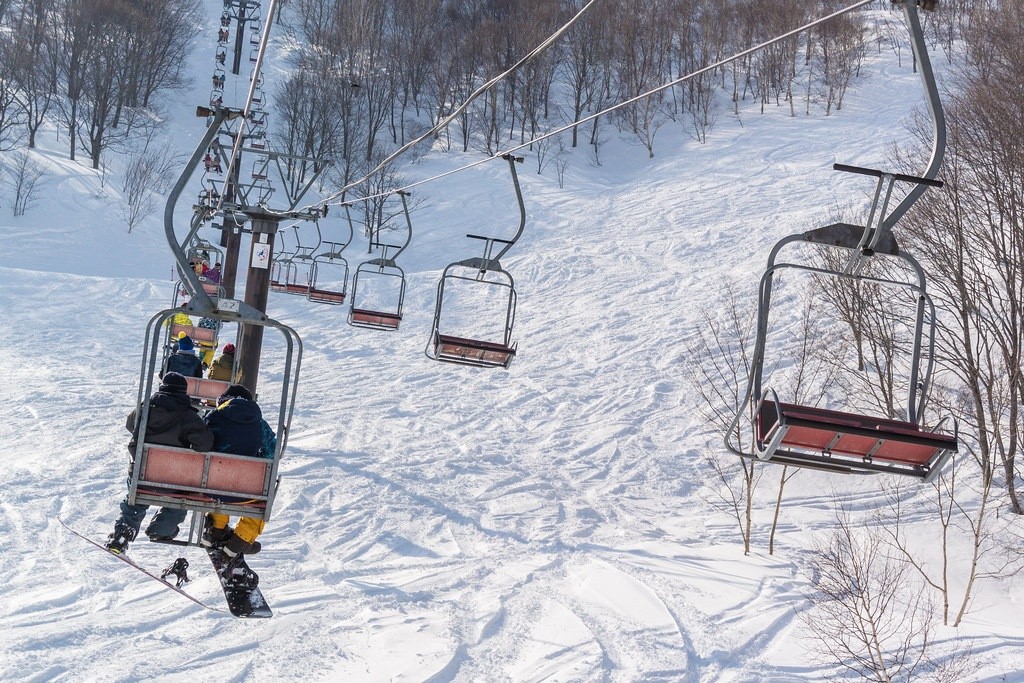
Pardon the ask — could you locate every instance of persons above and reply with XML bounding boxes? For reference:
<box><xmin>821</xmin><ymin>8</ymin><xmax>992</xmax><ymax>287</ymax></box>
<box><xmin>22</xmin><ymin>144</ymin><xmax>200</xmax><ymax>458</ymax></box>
<box><xmin>188</xmin><ymin>250</ymin><xmax>221</xmax><ymax>286</ymax></box>
<box><xmin>201</xmin><ymin>383</ymin><xmax>278</xmax><ymax>554</ymax></box>
<box><xmin>205</xmin><ymin>342</ymin><xmax>236</xmax><ymax>416</ymax></box>
<box><xmin>162</xmin><ymin>303</ymin><xmax>192</xmax><ymax>350</ymax></box>
<box><xmin>197</xmin><ymin>316</ymin><xmax>224</xmax><ymax>370</ymax></box>
<box><xmin>115</xmin><ymin>371</ymin><xmax>203</xmax><ymax>538</ymax></box>
<box><xmin>158</xmin><ymin>331</ymin><xmax>202</xmax><ymax>404</ymax></box>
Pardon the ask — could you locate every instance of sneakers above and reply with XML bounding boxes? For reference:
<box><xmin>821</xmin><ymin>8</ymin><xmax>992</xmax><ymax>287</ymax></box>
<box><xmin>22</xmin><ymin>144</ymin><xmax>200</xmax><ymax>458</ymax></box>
<box><xmin>223</xmin><ymin>533</ymin><xmax>262</xmax><ymax>558</ymax></box>
<box><xmin>145</xmin><ymin>525</ymin><xmax>180</xmax><ymax>541</ymax></box>
<box><xmin>201</xmin><ymin>526</ymin><xmax>235</xmax><ymax>546</ymax></box>
<box><xmin>105</xmin><ymin>524</ymin><xmax>135</xmax><ymax>552</ymax></box>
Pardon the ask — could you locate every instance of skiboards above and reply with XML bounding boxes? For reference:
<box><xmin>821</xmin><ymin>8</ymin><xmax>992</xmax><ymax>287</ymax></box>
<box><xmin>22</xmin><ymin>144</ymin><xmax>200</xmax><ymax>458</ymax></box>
<box><xmin>55</xmin><ymin>519</ymin><xmax>262</xmax><ymax>614</ymax></box>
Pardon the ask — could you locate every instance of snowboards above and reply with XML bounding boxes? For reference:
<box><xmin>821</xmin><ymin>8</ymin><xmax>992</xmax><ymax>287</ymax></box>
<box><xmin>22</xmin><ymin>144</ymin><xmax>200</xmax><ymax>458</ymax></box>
<box><xmin>204</xmin><ymin>525</ymin><xmax>276</xmax><ymax>617</ymax></box>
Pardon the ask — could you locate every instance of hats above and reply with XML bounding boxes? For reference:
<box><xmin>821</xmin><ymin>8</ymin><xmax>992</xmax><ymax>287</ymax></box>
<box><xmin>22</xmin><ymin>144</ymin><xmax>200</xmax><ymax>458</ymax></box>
<box><xmin>177</xmin><ymin>331</ymin><xmax>194</xmax><ymax>350</ymax></box>
<box><xmin>163</xmin><ymin>372</ymin><xmax>188</xmax><ymax>388</ymax></box>
<box><xmin>223</xmin><ymin>343</ymin><xmax>235</xmax><ymax>353</ymax></box>
<box><xmin>224</xmin><ymin>384</ymin><xmax>252</xmax><ymax>400</ymax></box>
